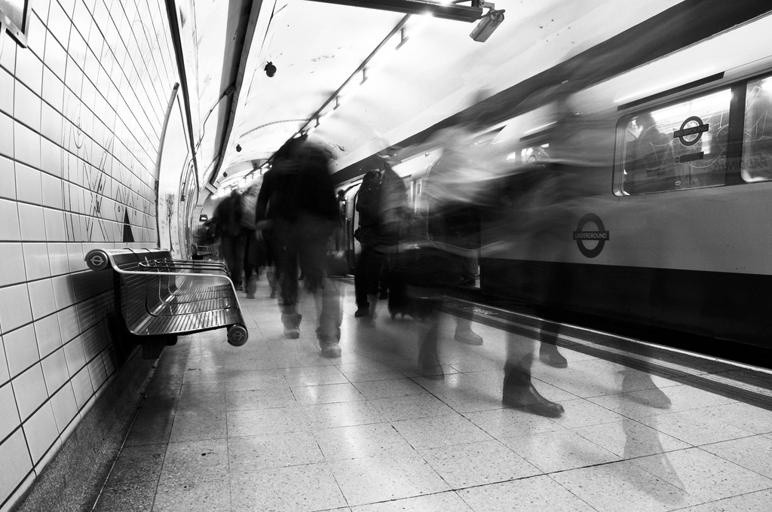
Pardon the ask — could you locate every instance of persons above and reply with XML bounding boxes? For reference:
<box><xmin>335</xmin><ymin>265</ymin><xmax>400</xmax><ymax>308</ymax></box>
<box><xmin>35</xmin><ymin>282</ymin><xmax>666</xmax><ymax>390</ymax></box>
<box><xmin>744</xmin><ymin>79</ymin><xmax>772</xmax><ymax>179</ymax></box>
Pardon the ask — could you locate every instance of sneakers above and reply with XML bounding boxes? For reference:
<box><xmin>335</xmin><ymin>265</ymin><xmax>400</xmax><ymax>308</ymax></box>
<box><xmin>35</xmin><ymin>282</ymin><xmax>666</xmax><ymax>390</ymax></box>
<box><xmin>321</xmin><ymin>342</ymin><xmax>340</xmax><ymax>358</ymax></box>
<box><xmin>236</xmin><ymin>284</ymin><xmax>255</xmax><ymax>299</ymax></box>
<box><xmin>283</xmin><ymin>326</ymin><xmax>300</xmax><ymax>338</ymax></box>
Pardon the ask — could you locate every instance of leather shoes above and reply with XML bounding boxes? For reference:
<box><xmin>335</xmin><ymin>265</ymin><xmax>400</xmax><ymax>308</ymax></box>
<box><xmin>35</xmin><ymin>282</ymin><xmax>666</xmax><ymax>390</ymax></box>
<box><xmin>619</xmin><ymin>371</ymin><xmax>671</xmax><ymax>408</ymax></box>
<box><xmin>420</xmin><ymin>355</ymin><xmax>444</xmax><ymax>379</ymax></box>
<box><xmin>453</xmin><ymin>329</ymin><xmax>485</xmax><ymax>345</ymax></box>
<box><xmin>625</xmin><ymin>441</ymin><xmax>683</xmax><ymax>498</ymax></box>
<box><xmin>503</xmin><ymin>382</ymin><xmax>564</xmax><ymax>416</ymax></box>
<box><xmin>540</xmin><ymin>348</ymin><xmax>569</xmax><ymax>369</ymax></box>
<box><xmin>354</xmin><ymin>306</ymin><xmax>369</xmax><ymax>317</ymax></box>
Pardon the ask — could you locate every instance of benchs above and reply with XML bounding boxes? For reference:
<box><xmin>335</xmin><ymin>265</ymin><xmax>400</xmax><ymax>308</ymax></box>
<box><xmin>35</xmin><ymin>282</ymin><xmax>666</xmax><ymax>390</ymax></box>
<box><xmin>84</xmin><ymin>247</ymin><xmax>249</xmax><ymax>360</ymax></box>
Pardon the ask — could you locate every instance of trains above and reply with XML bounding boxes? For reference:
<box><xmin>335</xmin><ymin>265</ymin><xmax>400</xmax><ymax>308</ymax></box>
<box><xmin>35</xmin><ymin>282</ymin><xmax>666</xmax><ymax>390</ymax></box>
<box><xmin>332</xmin><ymin>0</ymin><xmax>771</xmax><ymax>352</ymax></box>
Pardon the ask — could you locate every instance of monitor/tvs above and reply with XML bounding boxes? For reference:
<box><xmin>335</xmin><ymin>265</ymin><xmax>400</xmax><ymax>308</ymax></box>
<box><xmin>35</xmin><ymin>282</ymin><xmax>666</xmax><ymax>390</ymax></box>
<box><xmin>0</xmin><ymin>0</ymin><xmax>32</xmax><ymax>48</ymax></box>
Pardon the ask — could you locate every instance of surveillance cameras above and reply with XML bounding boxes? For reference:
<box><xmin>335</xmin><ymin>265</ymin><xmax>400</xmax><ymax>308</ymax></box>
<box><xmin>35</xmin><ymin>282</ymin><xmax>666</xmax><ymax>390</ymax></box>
<box><xmin>469</xmin><ymin>10</ymin><xmax>504</xmax><ymax>42</ymax></box>
